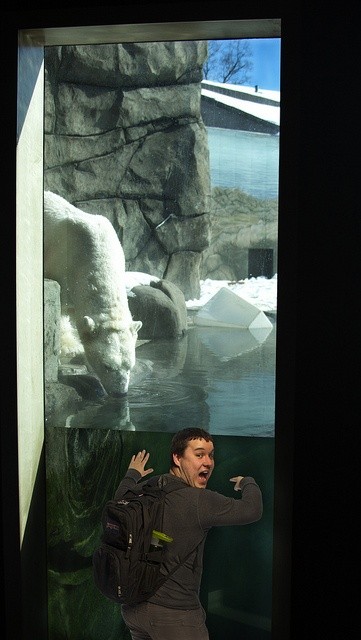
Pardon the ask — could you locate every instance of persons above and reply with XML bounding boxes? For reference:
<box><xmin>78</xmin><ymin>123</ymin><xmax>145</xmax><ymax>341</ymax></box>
<box><xmin>109</xmin><ymin>427</ymin><xmax>265</xmax><ymax>640</ymax></box>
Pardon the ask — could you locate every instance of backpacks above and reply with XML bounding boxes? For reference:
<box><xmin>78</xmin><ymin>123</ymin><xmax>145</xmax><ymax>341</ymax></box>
<box><xmin>93</xmin><ymin>475</ymin><xmax>207</xmax><ymax>605</ymax></box>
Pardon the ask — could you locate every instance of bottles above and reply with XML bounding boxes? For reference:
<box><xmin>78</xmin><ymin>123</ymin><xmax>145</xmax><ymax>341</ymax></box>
<box><xmin>150</xmin><ymin>531</ymin><xmax>172</xmax><ymax>564</ymax></box>
